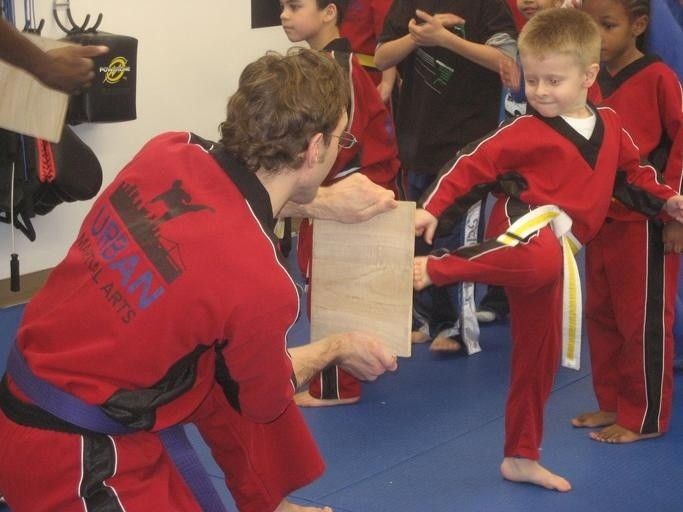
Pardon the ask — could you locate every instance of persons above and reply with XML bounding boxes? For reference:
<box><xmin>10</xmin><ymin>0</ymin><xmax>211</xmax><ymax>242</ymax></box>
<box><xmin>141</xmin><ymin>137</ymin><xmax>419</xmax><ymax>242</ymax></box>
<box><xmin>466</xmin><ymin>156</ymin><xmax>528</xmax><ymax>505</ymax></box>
<box><xmin>410</xmin><ymin>7</ymin><xmax>683</xmax><ymax>492</ymax></box>
<box><xmin>277</xmin><ymin>0</ymin><xmax>406</xmax><ymax>407</ymax></box>
<box><xmin>0</xmin><ymin>12</ymin><xmax>109</xmax><ymax>96</ymax></box>
<box><xmin>337</xmin><ymin>0</ymin><xmax>397</xmax><ymax>104</ymax></box>
<box><xmin>473</xmin><ymin>0</ymin><xmax>564</xmax><ymax>324</ymax></box>
<box><xmin>0</xmin><ymin>46</ymin><xmax>400</xmax><ymax>512</ymax></box>
<box><xmin>570</xmin><ymin>1</ymin><xmax>683</xmax><ymax>443</ymax></box>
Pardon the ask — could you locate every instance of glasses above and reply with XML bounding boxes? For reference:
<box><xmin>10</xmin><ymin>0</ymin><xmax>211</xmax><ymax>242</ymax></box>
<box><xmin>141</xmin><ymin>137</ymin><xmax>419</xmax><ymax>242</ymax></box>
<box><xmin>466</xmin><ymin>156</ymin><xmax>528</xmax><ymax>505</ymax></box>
<box><xmin>322</xmin><ymin>131</ymin><xmax>359</xmax><ymax>150</ymax></box>
